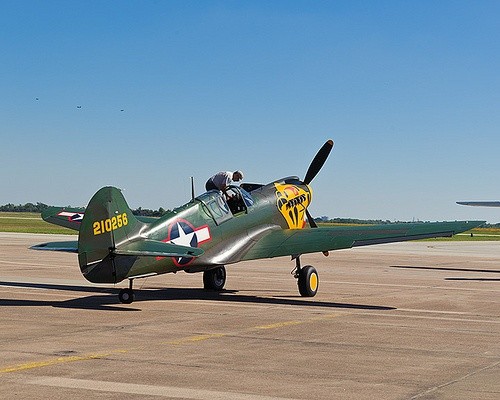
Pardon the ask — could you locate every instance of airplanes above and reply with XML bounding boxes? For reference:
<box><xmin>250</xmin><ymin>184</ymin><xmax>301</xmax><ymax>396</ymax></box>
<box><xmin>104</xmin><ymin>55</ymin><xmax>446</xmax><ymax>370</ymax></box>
<box><xmin>30</xmin><ymin>139</ymin><xmax>491</xmax><ymax>306</ymax></box>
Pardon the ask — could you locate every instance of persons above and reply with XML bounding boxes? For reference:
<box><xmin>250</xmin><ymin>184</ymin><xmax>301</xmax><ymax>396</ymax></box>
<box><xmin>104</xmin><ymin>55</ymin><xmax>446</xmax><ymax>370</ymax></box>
<box><xmin>206</xmin><ymin>170</ymin><xmax>243</xmax><ymax>192</ymax></box>
